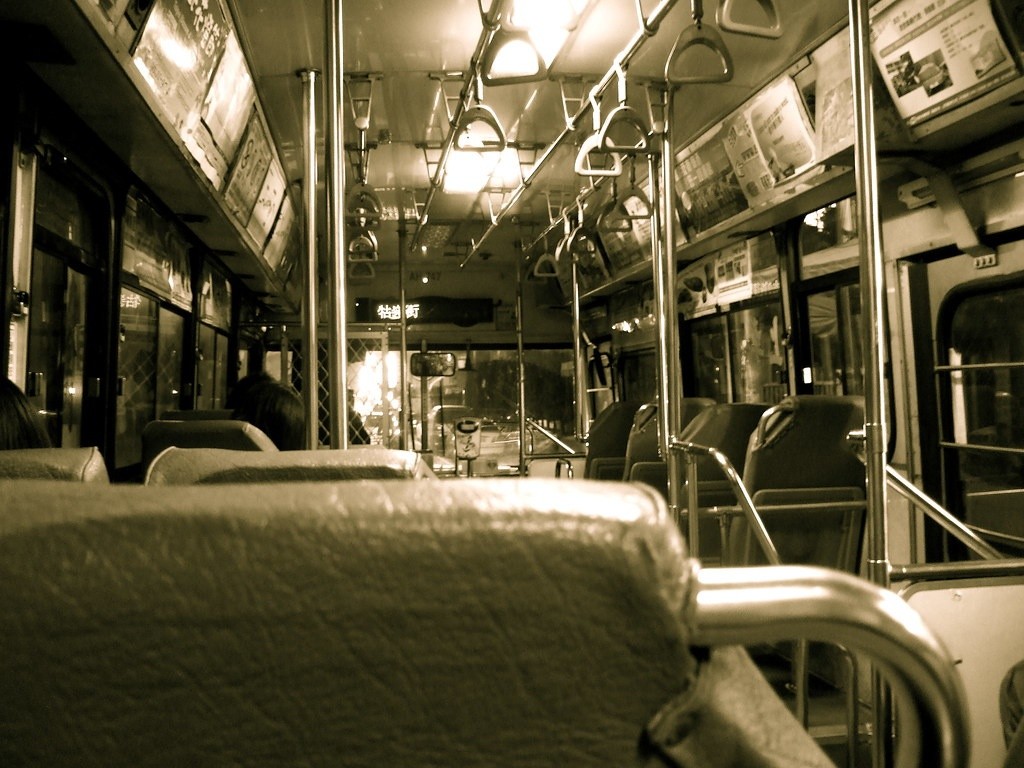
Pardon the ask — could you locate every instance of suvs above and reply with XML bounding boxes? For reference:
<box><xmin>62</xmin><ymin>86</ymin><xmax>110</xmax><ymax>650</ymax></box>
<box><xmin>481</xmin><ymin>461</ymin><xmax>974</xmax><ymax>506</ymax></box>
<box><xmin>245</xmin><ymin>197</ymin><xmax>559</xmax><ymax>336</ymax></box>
<box><xmin>412</xmin><ymin>405</ymin><xmax>477</xmax><ymax>447</ymax></box>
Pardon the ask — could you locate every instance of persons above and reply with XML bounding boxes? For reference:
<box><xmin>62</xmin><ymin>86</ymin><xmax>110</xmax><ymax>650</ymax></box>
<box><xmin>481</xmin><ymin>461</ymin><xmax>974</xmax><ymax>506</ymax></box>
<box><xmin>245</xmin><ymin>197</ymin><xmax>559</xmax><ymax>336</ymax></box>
<box><xmin>225</xmin><ymin>370</ymin><xmax>374</xmax><ymax>456</ymax></box>
<box><xmin>0</xmin><ymin>376</ymin><xmax>53</xmax><ymax>450</ymax></box>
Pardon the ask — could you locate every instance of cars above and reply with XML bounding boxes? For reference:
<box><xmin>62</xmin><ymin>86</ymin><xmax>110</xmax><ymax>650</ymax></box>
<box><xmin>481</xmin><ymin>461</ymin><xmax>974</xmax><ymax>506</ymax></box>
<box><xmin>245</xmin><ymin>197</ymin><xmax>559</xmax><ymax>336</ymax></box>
<box><xmin>360</xmin><ymin>398</ymin><xmax>421</xmax><ymax>436</ymax></box>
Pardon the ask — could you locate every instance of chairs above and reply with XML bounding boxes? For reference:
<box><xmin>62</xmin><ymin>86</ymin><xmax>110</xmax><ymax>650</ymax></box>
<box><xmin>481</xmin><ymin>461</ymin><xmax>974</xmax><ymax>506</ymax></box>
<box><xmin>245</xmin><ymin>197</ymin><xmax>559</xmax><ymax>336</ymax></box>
<box><xmin>0</xmin><ymin>397</ymin><xmax>974</xmax><ymax>768</ymax></box>
